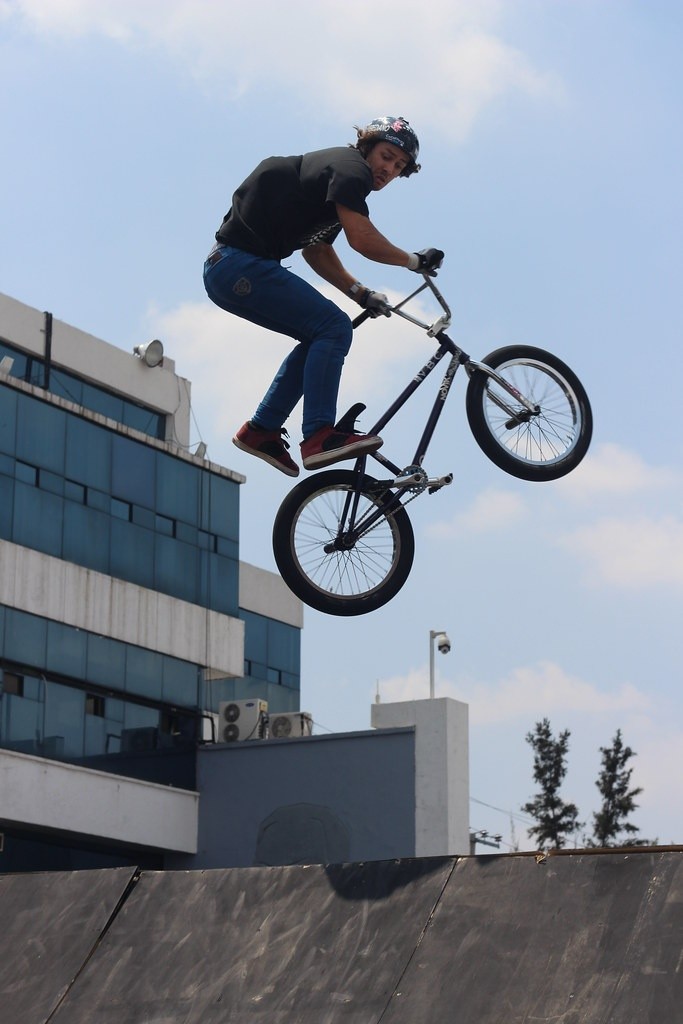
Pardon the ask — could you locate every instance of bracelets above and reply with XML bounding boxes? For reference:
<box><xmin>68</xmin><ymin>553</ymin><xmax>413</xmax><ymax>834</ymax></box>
<box><xmin>346</xmin><ymin>282</ymin><xmax>363</xmax><ymax>299</ymax></box>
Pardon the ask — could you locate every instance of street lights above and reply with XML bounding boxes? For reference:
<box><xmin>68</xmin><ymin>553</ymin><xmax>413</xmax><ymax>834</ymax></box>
<box><xmin>429</xmin><ymin>629</ymin><xmax>451</xmax><ymax>698</ymax></box>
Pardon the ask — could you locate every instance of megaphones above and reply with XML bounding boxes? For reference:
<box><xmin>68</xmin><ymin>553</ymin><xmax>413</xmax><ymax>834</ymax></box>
<box><xmin>134</xmin><ymin>339</ymin><xmax>164</xmax><ymax>368</ymax></box>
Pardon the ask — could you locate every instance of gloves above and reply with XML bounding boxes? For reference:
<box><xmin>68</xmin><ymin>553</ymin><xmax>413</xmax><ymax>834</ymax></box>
<box><xmin>358</xmin><ymin>288</ymin><xmax>392</xmax><ymax>319</ymax></box>
<box><xmin>407</xmin><ymin>248</ymin><xmax>445</xmax><ymax>277</ymax></box>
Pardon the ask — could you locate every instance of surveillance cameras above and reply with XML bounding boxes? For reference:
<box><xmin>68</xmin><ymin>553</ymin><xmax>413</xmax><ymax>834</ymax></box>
<box><xmin>438</xmin><ymin>637</ymin><xmax>450</xmax><ymax>654</ymax></box>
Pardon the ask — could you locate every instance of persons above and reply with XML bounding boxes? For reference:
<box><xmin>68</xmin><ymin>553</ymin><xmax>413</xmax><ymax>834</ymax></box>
<box><xmin>202</xmin><ymin>116</ymin><xmax>445</xmax><ymax>477</ymax></box>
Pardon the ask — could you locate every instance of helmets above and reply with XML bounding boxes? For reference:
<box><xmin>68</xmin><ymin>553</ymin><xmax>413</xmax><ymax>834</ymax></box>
<box><xmin>365</xmin><ymin>117</ymin><xmax>419</xmax><ymax>163</ymax></box>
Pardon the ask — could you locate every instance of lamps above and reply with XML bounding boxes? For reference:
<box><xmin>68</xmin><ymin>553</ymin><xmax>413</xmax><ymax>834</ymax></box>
<box><xmin>133</xmin><ymin>339</ymin><xmax>163</xmax><ymax>367</ymax></box>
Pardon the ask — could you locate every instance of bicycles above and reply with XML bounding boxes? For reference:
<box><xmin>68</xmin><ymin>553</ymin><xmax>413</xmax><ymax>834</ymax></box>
<box><xmin>272</xmin><ymin>249</ymin><xmax>592</xmax><ymax>617</ymax></box>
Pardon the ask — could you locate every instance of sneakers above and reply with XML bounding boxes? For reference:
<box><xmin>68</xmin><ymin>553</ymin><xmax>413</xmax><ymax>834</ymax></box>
<box><xmin>298</xmin><ymin>423</ymin><xmax>383</xmax><ymax>470</ymax></box>
<box><xmin>232</xmin><ymin>421</ymin><xmax>299</xmax><ymax>478</ymax></box>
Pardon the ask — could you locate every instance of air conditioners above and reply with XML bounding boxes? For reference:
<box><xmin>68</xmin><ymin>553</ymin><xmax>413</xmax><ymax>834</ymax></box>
<box><xmin>218</xmin><ymin>698</ymin><xmax>268</xmax><ymax>743</ymax></box>
<box><xmin>268</xmin><ymin>712</ymin><xmax>312</xmax><ymax>739</ymax></box>
